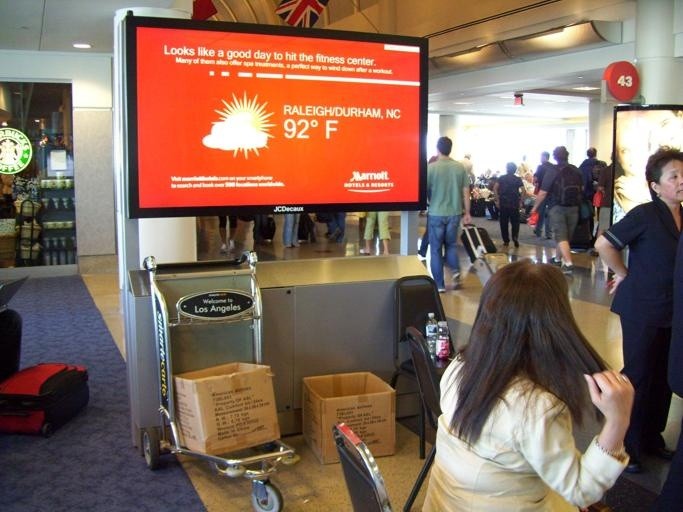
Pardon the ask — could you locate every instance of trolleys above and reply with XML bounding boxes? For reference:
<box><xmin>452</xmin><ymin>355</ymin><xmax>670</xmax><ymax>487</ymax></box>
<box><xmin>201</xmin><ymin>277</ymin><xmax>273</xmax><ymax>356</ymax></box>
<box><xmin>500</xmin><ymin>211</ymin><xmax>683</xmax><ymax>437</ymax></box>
<box><xmin>141</xmin><ymin>252</ymin><xmax>300</xmax><ymax>507</ymax></box>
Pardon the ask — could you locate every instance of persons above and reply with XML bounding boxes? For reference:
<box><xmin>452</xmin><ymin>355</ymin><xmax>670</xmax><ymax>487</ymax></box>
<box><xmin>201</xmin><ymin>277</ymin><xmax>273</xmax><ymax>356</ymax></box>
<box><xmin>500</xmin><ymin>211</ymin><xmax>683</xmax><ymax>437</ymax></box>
<box><xmin>417</xmin><ymin>159</ymin><xmax>449</xmax><ymax>261</ymax></box>
<box><xmin>423</xmin><ymin>135</ymin><xmax>474</xmax><ymax>294</ymax></box>
<box><xmin>594</xmin><ymin>146</ymin><xmax>683</xmax><ymax>475</ymax></box>
<box><xmin>219</xmin><ymin>213</ymin><xmax>390</xmax><ymax>255</ymax></box>
<box><xmin>645</xmin><ymin>228</ymin><xmax>683</xmax><ymax>512</ymax></box>
<box><xmin>423</xmin><ymin>255</ymin><xmax>638</xmax><ymax>512</ymax></box>
<box><xmin>461</xmin><ymin>146</ymin><xmax>614</xmax><ymax>273</ymax></box>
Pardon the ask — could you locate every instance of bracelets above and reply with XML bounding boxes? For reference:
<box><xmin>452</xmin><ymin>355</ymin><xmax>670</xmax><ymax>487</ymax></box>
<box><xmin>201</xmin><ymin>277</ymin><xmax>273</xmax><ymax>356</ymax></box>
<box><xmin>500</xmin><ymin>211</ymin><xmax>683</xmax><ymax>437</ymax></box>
<box><xmin>595</xmin><ymin>435</ymin><xmax>626</xmax><ymax>459</ymax></box>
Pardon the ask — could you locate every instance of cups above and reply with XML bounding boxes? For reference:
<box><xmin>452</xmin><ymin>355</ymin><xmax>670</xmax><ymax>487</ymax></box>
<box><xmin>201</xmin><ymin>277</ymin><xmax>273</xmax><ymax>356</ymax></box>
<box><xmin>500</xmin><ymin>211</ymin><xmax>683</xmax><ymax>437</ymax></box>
<box><xmin>38</xmin><ymin>178</ymin><xmax>74</xmax><ymax>264</ymax></box>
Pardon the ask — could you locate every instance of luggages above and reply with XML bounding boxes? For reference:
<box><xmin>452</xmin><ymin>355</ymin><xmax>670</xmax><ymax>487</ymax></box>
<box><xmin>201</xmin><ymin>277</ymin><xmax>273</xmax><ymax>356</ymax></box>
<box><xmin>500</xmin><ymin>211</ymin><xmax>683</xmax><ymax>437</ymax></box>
<box><xmin>567</xmin><ymin>199</ymin><xmax>595</xmax><ymax>251</ymax></box>
<box><xmin>0</xmin><ymin>363</ymin><xmax>89</xmax><ymax>438</ymax></box>
<box><xmin>461</xmin><ymin>224</ymin><xmax>496</xmax><ymax>263</ymax></box>
<box><xmin>462</xmin><ymin>224</ymin><xmax>509</xmax><ymax>288</ymax></box>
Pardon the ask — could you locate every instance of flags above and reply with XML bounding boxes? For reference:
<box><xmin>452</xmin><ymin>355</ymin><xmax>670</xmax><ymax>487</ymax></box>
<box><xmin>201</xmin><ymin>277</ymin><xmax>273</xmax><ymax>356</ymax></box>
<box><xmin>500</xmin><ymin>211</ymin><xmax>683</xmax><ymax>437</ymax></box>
<box><xmin>274</xmin><ymin>0</ymin><xmax>330</xmax><ymax>28</ymax></box>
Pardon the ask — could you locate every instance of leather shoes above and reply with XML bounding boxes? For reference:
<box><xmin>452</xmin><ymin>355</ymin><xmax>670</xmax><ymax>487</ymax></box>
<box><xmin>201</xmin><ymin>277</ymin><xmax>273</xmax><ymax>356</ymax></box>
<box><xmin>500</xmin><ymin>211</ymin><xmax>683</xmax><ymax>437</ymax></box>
<box><xmin>627</xmin><ymin>448</ymin><xmax>639</xmax><ymax>472</ymax></box>
<box><xmin>503</xmin><ymin>237</ymin><xmax>519</xmax><ymax>248</ymax></box>
<box><xmin>647</xmin><ymin>447</ymin><xmax>674</xmax><ymax>459</ymax></box>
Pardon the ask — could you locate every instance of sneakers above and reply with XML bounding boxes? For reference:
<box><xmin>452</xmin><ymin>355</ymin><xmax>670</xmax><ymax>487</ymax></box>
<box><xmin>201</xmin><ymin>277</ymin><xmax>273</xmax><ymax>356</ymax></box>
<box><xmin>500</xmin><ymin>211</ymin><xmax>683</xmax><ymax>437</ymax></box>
<box><xmin>221</xmin><ymin>240</ymin><xmax>235</xmax><ymax>250</ymax></box>
<box><xmin>590</xmin><ymin>251</ymin><xmax>598</xmax><ymax>257</ymax></box>
<box><xmin>417</xmin><ymin>253</ymin><xmax>426</xmax><ymax>262</ymax></box>
<box><xmin>438</xmin><ymin>272</ymin><xmax>461</xmax><ymax>293</ymax></box>
<box><xmin>549</xmin><ymin>258</ymin><xmax>561</xmax><ymax>267</ymax></box>
<box><xmin>562</xmin><ymin>264</ymin><xmax>574</xmax><ymax>274</ymax></box>
<box><xmin>329</xmin><ymin>227</ymin><xmax>341</xmax><ymax>240</ymax></box>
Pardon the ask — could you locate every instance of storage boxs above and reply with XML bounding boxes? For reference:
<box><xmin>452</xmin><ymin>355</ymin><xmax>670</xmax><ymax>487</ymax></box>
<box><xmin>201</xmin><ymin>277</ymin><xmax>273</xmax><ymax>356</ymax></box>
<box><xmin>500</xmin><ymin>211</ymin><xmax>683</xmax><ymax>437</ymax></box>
<box><xmin>171</xmin><ymin>361</ymin><xmax>281</xmax><ymax>456</ymax></box>
<box><xmin>302</xmin><ymin>370</ymin><xmax>398</xmax><ymax>465</ymax></box>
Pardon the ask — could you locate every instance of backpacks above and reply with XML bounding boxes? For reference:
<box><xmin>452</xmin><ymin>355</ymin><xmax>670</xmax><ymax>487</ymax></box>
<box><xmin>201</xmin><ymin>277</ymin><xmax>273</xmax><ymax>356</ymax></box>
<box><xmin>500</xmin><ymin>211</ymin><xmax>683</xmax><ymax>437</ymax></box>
<box><xmin>553</xmin><ymin>165</ymin><xmax>585</xmax><ymax>206</ymax></box>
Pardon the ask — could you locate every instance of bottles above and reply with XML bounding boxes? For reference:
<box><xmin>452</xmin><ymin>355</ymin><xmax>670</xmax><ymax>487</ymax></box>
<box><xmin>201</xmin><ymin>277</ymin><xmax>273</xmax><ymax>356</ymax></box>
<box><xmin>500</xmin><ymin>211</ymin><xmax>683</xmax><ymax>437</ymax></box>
<box><xmin>436</xmin><ymin>321</ymin><xmax>449</xmax><ymax>358</ymax></box>
<box><xmin>425</xmin><ymin>312</ymin><xmax>437</xmax><ymax>355</ymax></box>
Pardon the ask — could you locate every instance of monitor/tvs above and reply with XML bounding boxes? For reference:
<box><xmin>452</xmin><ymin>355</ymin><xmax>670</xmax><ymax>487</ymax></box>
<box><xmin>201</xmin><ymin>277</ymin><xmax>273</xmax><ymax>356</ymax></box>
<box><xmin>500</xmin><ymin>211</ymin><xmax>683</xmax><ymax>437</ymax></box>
<box><xmin>116</xmin><ymin>11</ymin><xmax>427</xmax><ymax>219</ymax></box>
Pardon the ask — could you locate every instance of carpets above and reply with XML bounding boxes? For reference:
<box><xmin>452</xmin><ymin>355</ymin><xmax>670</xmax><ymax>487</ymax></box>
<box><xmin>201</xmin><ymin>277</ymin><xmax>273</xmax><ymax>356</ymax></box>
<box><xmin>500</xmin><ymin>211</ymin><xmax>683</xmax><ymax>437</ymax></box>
<box><xmin>0</xmin><ymin>274</ymin><xmax>209</xmax><ymax>512</ymax></box>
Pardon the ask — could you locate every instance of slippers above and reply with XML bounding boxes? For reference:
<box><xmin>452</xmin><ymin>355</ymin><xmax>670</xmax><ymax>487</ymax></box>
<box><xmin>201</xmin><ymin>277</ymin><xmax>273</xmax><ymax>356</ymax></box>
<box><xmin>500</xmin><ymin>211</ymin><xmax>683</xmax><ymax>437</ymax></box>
<box><xmin>359</xmin><ymin>249</ymin><xmax>370</xmax><ymax>256</ymax></box>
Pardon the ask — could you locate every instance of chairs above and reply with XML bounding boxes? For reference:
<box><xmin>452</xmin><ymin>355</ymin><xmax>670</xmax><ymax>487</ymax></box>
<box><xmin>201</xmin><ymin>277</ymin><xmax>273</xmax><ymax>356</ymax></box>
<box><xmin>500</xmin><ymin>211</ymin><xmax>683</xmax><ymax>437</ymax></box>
<box><xmin>388</xmin><ymin>274</ymin><xmax>457</xmax><ymax>512</ymax></box>
